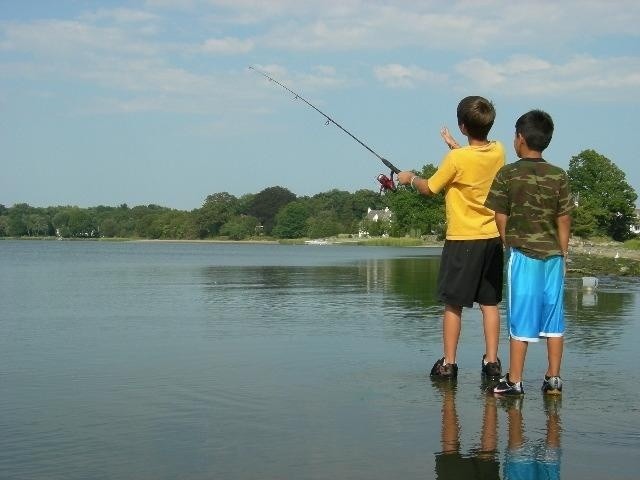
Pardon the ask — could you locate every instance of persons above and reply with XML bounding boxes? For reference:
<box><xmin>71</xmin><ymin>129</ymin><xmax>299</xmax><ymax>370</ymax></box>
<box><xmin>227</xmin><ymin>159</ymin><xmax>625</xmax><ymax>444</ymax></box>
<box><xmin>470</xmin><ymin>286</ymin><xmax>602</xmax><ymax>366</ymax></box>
<box><xmin>398</xmin><ymin>95</ymin><xmax>506</xmax><ymax>383</ymax></box>
<box><xmin>435</xmin><ymin>379</ymin><xmax>502</xmax><ymax>480</ymax></box>
<box><xmin>494</xmin><ymin>394</ymin><xmax>565</xmax><ymax>479</ymax></box>
<box><xmin>480</xmin><ymin>108</ymin><xmax>575</xmax><ymax>400</ymax></box>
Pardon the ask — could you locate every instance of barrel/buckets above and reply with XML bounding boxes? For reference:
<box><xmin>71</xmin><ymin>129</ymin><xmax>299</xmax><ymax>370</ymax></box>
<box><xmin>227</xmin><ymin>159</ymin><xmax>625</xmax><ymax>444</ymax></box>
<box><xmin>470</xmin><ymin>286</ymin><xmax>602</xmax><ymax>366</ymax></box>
<box><xmin>583</xmin><ymin>277</ymin><xmax>599</xmax><ymax>288</ymax></box>
<box><xmin>581</xmin><ymin>294</ymin><xmax>599</xmax><ymax>306</ymax></box>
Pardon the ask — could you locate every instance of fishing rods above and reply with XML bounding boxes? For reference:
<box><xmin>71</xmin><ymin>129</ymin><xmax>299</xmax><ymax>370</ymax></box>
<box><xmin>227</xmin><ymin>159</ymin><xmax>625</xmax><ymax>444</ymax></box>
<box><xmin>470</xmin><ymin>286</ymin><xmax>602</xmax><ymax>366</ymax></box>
<box><xmin>247</xmin><ymin>65</ymin><xmax>417</xmax><ymax>194</ymax></box>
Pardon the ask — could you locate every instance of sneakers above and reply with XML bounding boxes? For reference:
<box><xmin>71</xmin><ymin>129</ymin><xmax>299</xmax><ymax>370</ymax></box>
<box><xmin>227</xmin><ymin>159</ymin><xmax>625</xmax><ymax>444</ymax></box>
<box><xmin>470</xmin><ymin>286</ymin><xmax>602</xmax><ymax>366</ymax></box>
<box><xmin>540</xmin><ymin>372</ymin><xmax>563</xmax><ymax>396</ymax></box>
<box><xmin>429</xmin><ymin>356</ymin><xmax>459</xmax><ymax>380</ymax></box>
<box><xmin>486</xmin><ymin>372</ymin><xmax>526</xmax><ymax>397</ymax></box>
<box><xmin>481</xmin><ymin>353</ymin><xmax>503</xmax><ymax>378</ymax></box>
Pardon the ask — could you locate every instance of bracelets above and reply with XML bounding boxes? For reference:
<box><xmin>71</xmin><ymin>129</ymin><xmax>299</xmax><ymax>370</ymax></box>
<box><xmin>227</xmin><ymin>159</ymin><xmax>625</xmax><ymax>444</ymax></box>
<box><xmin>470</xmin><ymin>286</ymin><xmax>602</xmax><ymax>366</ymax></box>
<box><xmin>410</xmin><ymin>175</ymin><xmax>419</xmax><ymax>189</ymax></box>
<box><xmin>450</xmin><ymin>143</ymin><xmax>457</xmax><ymax>150</ymax></box>
